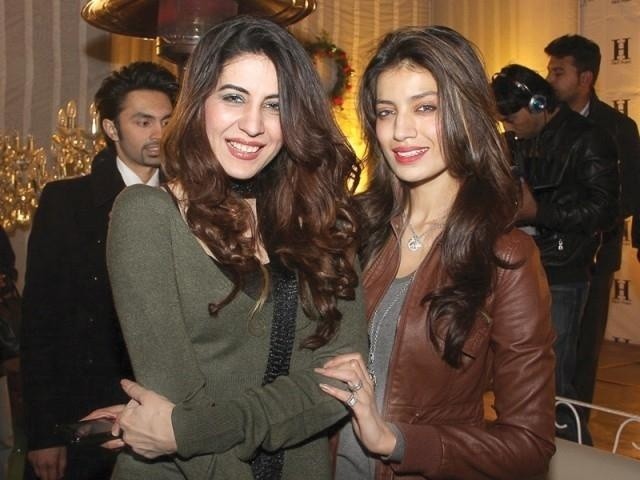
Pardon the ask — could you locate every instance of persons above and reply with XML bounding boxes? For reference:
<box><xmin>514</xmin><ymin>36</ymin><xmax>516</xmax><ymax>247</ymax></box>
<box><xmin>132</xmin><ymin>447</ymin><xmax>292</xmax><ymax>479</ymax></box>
<box><xmin>110</xmin><ymin>13</ymin><xmax>367</xmax><ymax>480</ymax></box>
<box><xmin>0</xmin><ymin>225</ymin><xmax>19</xmax><ymax>479</ymax></box>
<box><xmin>19</xmin><ymin>62</ymin><xmax>182</xmax><ymax>480</ymax></box>
<box><xmin>544</xmin><ymin>35</ymin><xmax>640</xmax><ymax>426</ymax></box>
<box><xmin>77</xmin><ymin>24</ymin><xmax>556</xmax><ymax>480</ymax></box>
<box><xmin>491</xmin><ymin>63</ymin><xmax>623</xmax><ymax>447</ymax></box>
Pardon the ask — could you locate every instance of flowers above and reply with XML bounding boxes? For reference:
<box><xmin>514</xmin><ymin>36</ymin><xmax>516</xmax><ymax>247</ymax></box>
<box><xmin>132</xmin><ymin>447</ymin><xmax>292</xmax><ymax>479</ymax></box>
<box><xmin>298</xmin><ymin>26</ymin><xmax>357</xmax><ymax>113</ymax></box>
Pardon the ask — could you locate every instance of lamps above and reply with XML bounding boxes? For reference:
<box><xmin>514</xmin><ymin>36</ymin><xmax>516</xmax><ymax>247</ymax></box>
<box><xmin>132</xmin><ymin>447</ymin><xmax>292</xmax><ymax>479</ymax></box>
<box><xmin>78</xmin><ymin>0</ymin><xmax>315</xmax><ymax>77</ymax></box>
<box><xmin>0</xmin><ymin>95</ymin><xmax>107</xmax><ymax>243</ymax></box>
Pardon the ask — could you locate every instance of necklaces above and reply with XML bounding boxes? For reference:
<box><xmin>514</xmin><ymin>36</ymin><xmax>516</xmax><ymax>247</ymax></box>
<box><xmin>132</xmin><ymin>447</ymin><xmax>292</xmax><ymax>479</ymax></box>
<box><xmin>402</xmin><ymin>213</ymin><xmax>437</xmax><ymax>252</ymax></box>
<box><xmin>367</xmin><ymin>277</ymin><xmax>411</xmax><ymax>390</ymax></box>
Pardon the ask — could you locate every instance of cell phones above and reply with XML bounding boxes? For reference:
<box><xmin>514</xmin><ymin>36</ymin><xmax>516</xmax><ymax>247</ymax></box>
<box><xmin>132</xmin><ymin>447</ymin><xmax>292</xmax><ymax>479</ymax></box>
<box><xmin>53</xmin><ymin>417</ymin><xmax>124</xmax><ymax>448</ymax></box>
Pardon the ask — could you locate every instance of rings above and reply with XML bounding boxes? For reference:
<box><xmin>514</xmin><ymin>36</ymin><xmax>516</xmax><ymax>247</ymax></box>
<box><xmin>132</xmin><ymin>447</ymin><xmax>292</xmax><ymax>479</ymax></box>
<box><xmin>345</xmin><ymin>394</ymin><xmax>358</xmax><ymax>408</ymax></box>
<box><xmin>349</xmin><ymin>382</ymin><xmax>363</xmax><ymax>391</ymax></box>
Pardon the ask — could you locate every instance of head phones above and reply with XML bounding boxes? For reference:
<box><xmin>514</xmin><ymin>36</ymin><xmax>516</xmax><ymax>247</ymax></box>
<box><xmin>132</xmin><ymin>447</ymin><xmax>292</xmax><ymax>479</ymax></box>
<box><xmin>492</xmin><ymin>73</ymin><xmax>548</xmax><ymax>115</ymax></box>
